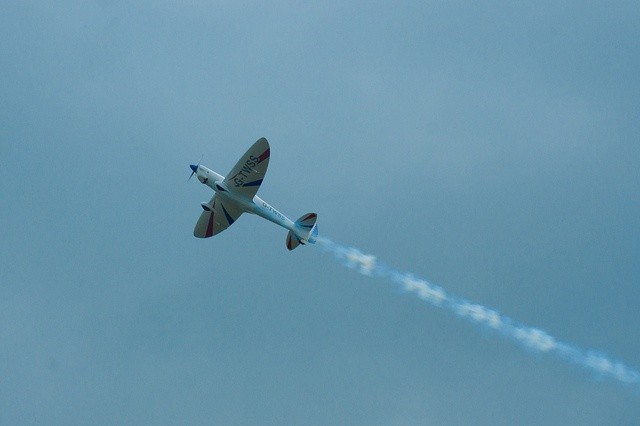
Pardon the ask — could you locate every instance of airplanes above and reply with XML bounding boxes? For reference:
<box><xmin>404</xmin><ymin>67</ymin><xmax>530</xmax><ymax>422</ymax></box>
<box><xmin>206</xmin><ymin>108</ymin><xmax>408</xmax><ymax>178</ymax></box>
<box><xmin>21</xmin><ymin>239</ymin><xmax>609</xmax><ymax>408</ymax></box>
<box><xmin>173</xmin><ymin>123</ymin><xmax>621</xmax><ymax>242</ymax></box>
<box><xmin>187</xmin><ymin>137</ymin><xmax>318</xmax><ymax>251</ymax></box>
